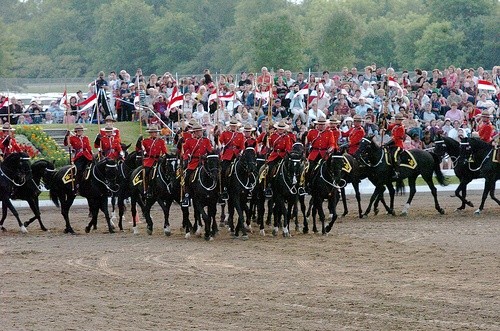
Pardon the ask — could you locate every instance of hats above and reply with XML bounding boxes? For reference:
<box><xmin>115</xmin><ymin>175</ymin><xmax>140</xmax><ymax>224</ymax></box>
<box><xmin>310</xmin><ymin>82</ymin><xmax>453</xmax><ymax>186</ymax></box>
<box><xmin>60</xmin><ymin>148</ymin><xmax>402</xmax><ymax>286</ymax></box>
<box><xmin>103</xmin><ymin>115</ymin><xmax>116</xmax><ymax>122</ymax></box>
<box><xmin>451</xmin><ymin>102</ymin><xmax>457</xmax><ymax>106</ymax></box>
<box><xmin>481</xmin><ymin>109</ymin><xmax>491</xmax><ymax>117</ymax></box>
<box><xmin>395</xmin><ymin>113</ymin><xmax>404</xmax><ymax>120</ymax></box>
<box><xmin>146</xmin><ymin>124</ymin><xmax>162</xmax><ymax>132</ymax></box>
<box><xmin>73</xmin><ymin>124</ymin><xmax>87</xmax><ymax>131</ymax></box>
<box><xmin>100</xmin><ymin>125</ymin><xmax>117</xmax><ymax>131</ymax></box>
<box><xmin>0</xmin><ymin>122</ymin><xmax>16</xmax><ymax>130</ymax></box>
<box><xmin>187</xmin><ymin>114</ymin><xmax>363</xmax><ymax>131</ymax></box>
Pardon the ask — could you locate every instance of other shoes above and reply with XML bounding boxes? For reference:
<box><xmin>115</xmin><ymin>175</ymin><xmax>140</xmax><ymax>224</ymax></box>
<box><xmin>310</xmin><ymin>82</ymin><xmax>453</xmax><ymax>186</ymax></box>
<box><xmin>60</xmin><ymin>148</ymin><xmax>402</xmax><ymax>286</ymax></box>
<box><xmin>266</xmin><ymin>188</ymin><xmax>272</xmax><ymax>198</ymax></box>
<box><xmin>222</xmin><ymin>191</ymin><xmax>229</xmax><ymax>201</ymax></box>
<box><xmin>182</xmin><ymin>198</ymin><xmax>188</xmax><ymax>207</ymax></box>
<box><xmin>246</xmin><ymin>190</ymin><xmax>253</xmax><ymax>199</ymax></box>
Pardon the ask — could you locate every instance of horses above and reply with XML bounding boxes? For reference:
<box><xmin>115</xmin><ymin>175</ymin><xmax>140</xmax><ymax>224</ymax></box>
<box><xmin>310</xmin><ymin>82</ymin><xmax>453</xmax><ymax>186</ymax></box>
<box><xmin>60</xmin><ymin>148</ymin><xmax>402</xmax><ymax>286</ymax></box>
<box><xmin>0</xmin><ymin>152</ymin><xmax>33</xmax><ymax>233</ymax></box>
<box><xmin>45</xmin><ymin>156</ymin><xmax>118</xmax><ymax>234</ymax></box>
<box><xmin>434</xmin><ymin>132</ymin><xmax>500</xmax><ymax>217</ymax></box>
<box><xmin>108</xmin><ymin>136</ymin><xmax>451</xmax><ymax>241</ymax></box>
<box><xmin>9</xmin><ymin>160</ymin><xmax>56</xmax><ymax>233</ymax></box>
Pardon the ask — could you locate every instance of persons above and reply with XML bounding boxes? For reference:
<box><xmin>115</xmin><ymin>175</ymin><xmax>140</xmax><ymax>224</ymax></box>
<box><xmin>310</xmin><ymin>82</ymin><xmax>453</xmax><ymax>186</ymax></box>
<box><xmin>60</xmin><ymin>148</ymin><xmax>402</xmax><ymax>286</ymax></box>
<box><xmin>0</xmin><ymin>63</ymin><xmax>500</xmax><ymax>206</ymax></box>
<box><xmin>383</xmin><ymin>113</ymin><xmax>406</xmax><ymax>176</ymax></box>
